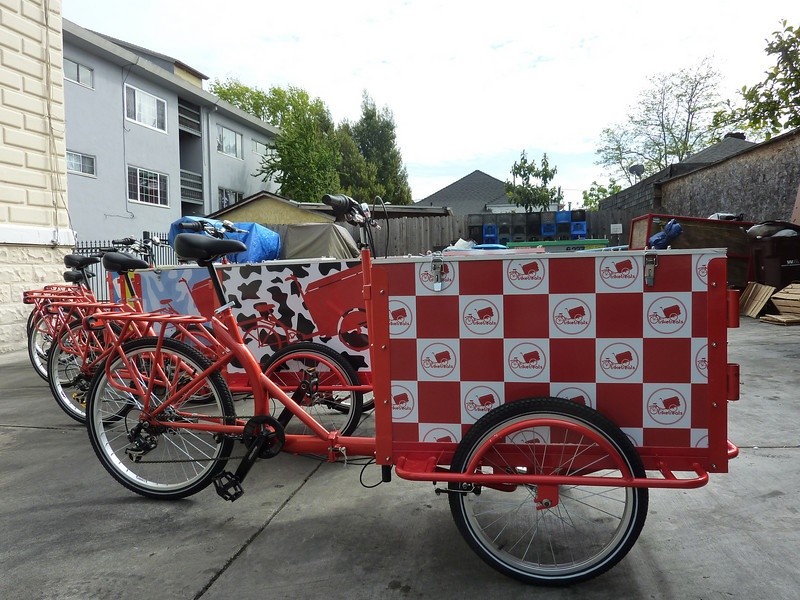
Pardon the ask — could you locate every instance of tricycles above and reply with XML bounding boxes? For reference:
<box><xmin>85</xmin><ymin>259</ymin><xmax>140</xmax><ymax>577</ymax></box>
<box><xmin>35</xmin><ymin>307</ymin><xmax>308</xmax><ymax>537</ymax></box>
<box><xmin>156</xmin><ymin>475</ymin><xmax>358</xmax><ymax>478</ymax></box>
<box><xmin>83</xmin><ymin>194</ymin><xmax>742</xmax><ymax>590</ymax></box>
<box><xmin>22</xmin><ymin>218</ymin><xmax>375</xmax><ymax>437</ymax></box>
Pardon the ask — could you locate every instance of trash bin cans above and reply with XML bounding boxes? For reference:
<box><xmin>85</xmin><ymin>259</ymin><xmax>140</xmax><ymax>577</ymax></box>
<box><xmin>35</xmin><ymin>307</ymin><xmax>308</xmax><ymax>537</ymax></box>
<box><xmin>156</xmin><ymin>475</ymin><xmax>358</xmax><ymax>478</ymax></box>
<box><xmin>749</xmin><ymin>220</ymin><xmax>800</xmax><ymax>314</ymax></box>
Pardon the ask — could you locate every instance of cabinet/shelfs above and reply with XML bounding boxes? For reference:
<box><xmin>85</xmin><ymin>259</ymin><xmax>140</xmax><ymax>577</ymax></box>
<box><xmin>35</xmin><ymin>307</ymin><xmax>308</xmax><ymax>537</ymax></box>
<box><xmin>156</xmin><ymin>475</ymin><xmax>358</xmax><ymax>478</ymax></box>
<box><xmin>762</xmin><ymin>236</ymin><xmax>800</xmax><ymax>315</ymax></box>
